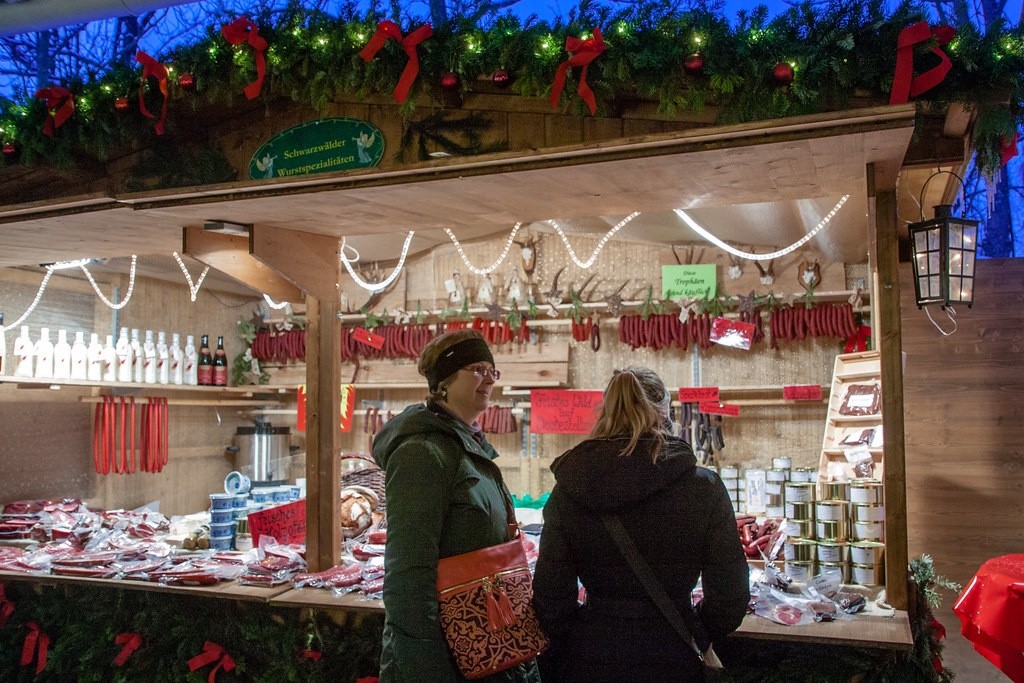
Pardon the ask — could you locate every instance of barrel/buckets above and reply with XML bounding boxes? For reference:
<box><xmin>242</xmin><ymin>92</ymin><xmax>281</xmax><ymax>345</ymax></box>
<box><xmin>227</xmin><ymin>423</ymin><xmax>299</xmax><ymax>485</ymax></box>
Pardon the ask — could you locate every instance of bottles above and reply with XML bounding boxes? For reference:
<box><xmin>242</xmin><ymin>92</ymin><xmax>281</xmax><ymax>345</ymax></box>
<box><xmin>86</xmin><ymin>333</ymin><xmax>104</xmax><ymax>380</ymax></box>
<box><xmin>103</xmin><ymin>335</ymin><xmax>118</xmax><ymax>382</ymax></box>
<box><xmin>143</xmin><ymin>329</ymin><xmax>228</xmax><ymax>386</ymax></box>
<box><xmin>36</xmin><ymin>326</ymin><xmax>54</xmax><ymax>378</ymax></box>
<box><xmin>129</xmin><ymin>329</ymin><xmax>144</xmax><ymax>382</ymax></box>
<box><xmin>116</xmin><ymin>326</ymin><xmax>133</xmax><ymax>382</ymax></box>
<box><xmin>0</xmin><ymin>313</ymin><xmax>5</xmax><ymax>375</ymax></box>
<box><xmin>705</xmin><ymin>455</ymin><xmax>886</xmax><ymax>588</ymax></box>
<box><xmin>53</xmin><ymin>330</ymin><xmax>72</xmax><ymax>379</ymax></box>
<box><xmin>448</xmin><ymin>270</ymin><xmax>528</xmax><ymax>307</ymax></box>
<box><xmin>13</xmin><ymin>324</ymin><xmax>33</xmax><ymax>377</ymax></box>
<box><xmin>71</xmin><ymin>332</ymin><xmax>89</xmax><ymax>381</ymax></box>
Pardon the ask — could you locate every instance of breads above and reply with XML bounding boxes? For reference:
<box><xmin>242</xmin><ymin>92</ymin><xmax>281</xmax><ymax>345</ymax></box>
<box><xmin>341</xmin><ymin>484</ymin><xmax>387</xmax><ymax>539</ymax></box>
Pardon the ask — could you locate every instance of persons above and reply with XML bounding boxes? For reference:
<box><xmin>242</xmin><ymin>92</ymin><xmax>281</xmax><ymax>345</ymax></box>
<box><xmin>530</xmin><ymin>365</ymin><xmax>750</xmax><ymax>683</ymax></box>
<box><xmin>371</xmin><ymin>328</ymin><xmax>543</xmax><ymax>683</ymax></box>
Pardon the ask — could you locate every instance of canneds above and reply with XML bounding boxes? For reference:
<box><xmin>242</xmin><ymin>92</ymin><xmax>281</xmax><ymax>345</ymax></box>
<box><xmin>703</xmin><ymin>457</ymin><xmax>884</xmax><ymax>542</ymax></box>
<box><xmin>184</xmin><ymin>524</ymin><xmax>210</xmax><ymax>550</ymax></box>
<box><xmin>782</xmin><ymin>537</ymin><xmax>885</xmax><ymax>588</ymax></box>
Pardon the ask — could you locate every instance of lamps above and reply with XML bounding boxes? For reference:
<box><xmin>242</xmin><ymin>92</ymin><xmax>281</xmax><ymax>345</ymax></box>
<box><xmin>897</xmin><ymin>167</ymin><xmax>981</xmax><ymax>308</ymax></box>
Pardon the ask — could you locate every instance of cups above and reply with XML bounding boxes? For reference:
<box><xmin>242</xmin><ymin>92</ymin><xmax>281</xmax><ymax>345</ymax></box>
<box><xmin>209</xmin><ymin>469</ymin><xmax>303</xmax><ymax>551</ymax></box>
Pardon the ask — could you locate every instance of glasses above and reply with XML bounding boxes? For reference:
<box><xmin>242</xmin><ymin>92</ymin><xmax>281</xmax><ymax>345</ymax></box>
<box><xmin>460</xmin><ymin>365</ymin><xmax>501</xmax><ymax>380</ymax></box>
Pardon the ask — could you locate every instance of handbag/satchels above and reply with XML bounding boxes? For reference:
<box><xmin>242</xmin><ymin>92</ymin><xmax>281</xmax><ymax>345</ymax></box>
<box><xmin>697</xmin><ymin>651</ymin><xmax>736</xmax><ymax>683</ymax></box>
<box><xmin>343</xmin><ymin>453</ymin><xmax>387</xmax><ymax>516</ymax></box>
<box><xmin>436</xmin><ymin>522</ymin><xmax>554</xmax><ymax>679</ymax></box>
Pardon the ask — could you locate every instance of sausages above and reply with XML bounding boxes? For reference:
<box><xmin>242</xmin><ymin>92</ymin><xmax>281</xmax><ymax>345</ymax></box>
<box><xmin>251</xmin><ymin>328</ymin><xmax>306</xmax><ymax>365</ymax></box>
<box><xmin>342</xmin><ymin>315</ymin><xmax>531</xmax><ymax>384</ymax></box>
<box><xmin>364</xmin><ymin>407</ymin><xmax>396</xmax><ymax>456</ymax></box>
<box><xmin>681</xmin><ymin>400</ymin><xmax>726</xmax><ymax>465</ymax></box>
<box><xmin>618</xmin><ymin>312</ymin><xmax>725</xmax><ymax>352</ymax></box>
<box><xmin>735</xmin><ymin>514</ymin><xmax>786</xmax><ymax>561</ymax></box>
<box><xmin>474</xmin><ymin>405</ymin><xmax>518</xmax><ymax>434</ymax></box>
<box><xmin>739</xmin><ymin>302</ymin><xmax>860</xmax><ymax>351</ymax></box>
<box><xmin>93</xmin><ymin>394</ymin><xmax>168</xmax><ymax>475</ymax></box>
<box><xmin>572</xmin><ymin>314</ymin><xmax>600</xmax><ymax>352</ymax></box>
